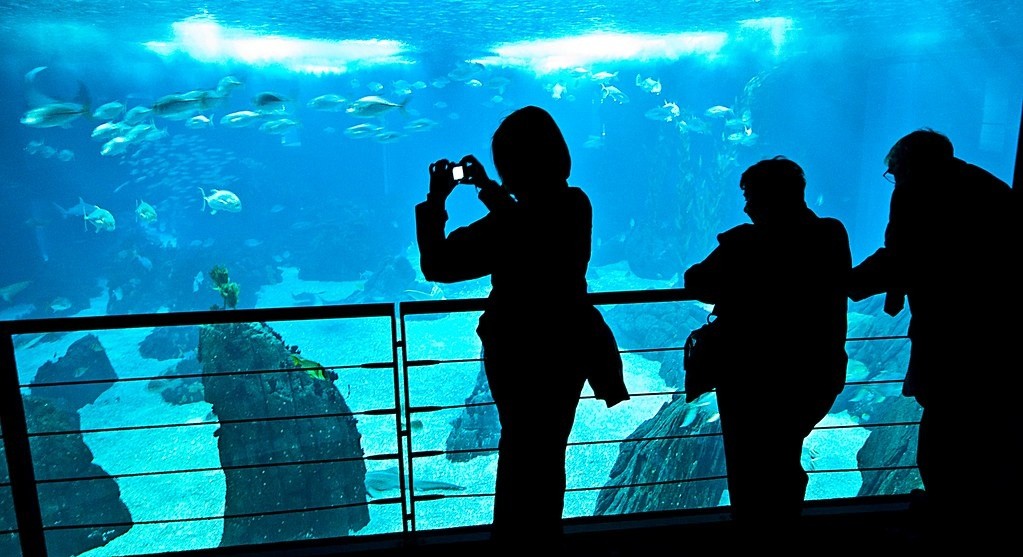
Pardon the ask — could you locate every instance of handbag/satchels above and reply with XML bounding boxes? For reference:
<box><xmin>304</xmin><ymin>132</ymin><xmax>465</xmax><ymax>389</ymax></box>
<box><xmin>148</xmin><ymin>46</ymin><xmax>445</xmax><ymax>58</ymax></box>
<box><xmin>580</xmin><ymin>302</ymin><xmax>630</xmax><ymax>410</ymax></box>
<box><xmin>682</xmin><ymin>314</ymin><xmax>717</xmax><ymax>402</ymax></box>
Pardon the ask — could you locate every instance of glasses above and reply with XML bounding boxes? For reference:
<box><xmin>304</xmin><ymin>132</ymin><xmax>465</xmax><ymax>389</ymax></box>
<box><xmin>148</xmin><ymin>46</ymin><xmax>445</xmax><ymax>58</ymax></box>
<box><xmin>882</xmin><ymin>162</ymin><xmax>896</xmax><ymax>185</ymax></box>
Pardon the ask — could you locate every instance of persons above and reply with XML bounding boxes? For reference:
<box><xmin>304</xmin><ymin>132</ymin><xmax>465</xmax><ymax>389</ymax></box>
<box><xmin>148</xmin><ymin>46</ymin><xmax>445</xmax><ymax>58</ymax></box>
<box><xmin>683</xmin><ymin>155</ymin><xmax>852</xmax><ymax>557</ymax></box>
<box><xmin>882</xmin><ymin>128</ymin><xmax>1023</xmax><ymax>556</ymax></box>
<box><xmin>414</xmin><ymin>108</ymin><xmax>593</xmax><ymax>557</ymax></box>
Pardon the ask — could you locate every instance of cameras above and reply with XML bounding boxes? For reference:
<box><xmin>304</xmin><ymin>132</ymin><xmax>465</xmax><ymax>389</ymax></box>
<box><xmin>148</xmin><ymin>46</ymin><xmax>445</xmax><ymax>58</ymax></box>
<box><xmin>448</xmin><ymin>163</ymin><xmax>469</xmax><ymax>182</ymax></box>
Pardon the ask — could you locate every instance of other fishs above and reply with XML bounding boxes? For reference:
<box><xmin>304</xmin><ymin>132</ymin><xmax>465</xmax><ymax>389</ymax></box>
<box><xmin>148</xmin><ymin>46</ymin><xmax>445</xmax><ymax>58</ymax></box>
<box><xmin>17</xmin><ymin>70</ymin><xmax>763</xmax><ymax>236</ymax></box>
<box><xmin>288</xmin><ymin>354</ymin><xmax>334</xmax><ymax>383</ymax></box>
<box><xmin>51</xmin><ymin>297</ymin><xmax>72</xmax><ymax>313</ymax></box>
